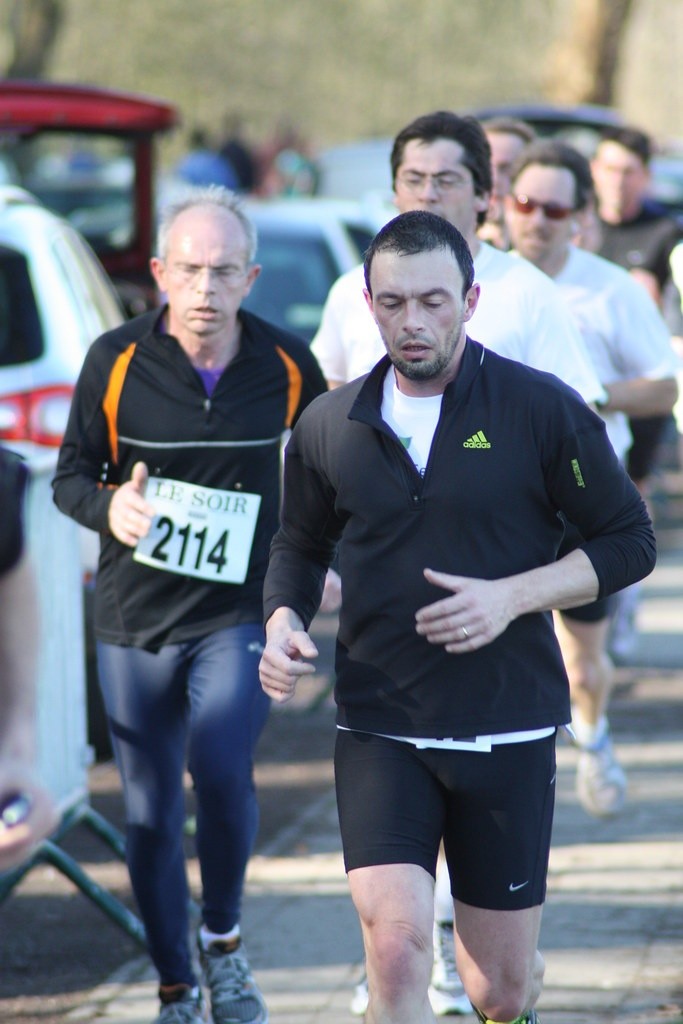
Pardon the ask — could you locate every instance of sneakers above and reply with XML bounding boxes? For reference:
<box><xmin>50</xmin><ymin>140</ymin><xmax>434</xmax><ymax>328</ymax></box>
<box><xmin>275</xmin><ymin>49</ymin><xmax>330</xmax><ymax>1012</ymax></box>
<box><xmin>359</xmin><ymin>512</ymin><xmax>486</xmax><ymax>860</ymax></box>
<box><xmin>427</xmin><ymin>916</ymin><xmax>471</xmax><ymax>1013</ymax></box>
<box><xmin>468</xmin><ymin>998</ymin><xmax>541</xmax><ymax>1024</ymax></box>
<box><xmin>351</xmin><ymin>980</ymin><xmax>368</xmax><ymax>1013</ymax></box>
<box><xmin>155</xmin><ymin>985</ymin><xmax>205</xmax><ymax>1024</ymax></box>
<box><xmin>196</xmin><ymin>919</ymin><xmax>267</xmax><ymax>1024</ymax></box>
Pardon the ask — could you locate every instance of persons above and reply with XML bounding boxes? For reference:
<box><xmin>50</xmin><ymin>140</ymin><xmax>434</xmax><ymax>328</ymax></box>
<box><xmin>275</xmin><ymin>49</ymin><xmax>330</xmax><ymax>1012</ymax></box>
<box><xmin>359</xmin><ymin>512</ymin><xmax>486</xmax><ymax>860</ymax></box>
<box><xmin>50</xmin><ymin>186</ymin><xmax>328</xmax><ymax>1024</ymax></box>
<box><xmin>308</xmin><ymin>112</ymin><xmax>683</xmax><ymax>819</ymax></box>
<box><xmin>259</xmin><ymin>209</ymin><xmax>657</xmax><ymax>1024</ymax></box>
<box><xmin>0</xmin><ymin>448</ymin><xmax>54</xmax><ymax>866</ymax></box>
<box><xmin>178</xmin><ymin>112</ymin><xmax>322</xmax><ymax>199</ymax></box>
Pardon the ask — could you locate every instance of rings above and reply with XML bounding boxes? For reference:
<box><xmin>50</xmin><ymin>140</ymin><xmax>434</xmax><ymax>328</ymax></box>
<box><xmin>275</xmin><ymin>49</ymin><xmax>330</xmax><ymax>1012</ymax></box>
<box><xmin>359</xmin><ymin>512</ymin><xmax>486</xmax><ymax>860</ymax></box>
<box><xmin>462</xmin><ymin>625</ymin><xmax>470</xmax><ymax>639</ymax></box>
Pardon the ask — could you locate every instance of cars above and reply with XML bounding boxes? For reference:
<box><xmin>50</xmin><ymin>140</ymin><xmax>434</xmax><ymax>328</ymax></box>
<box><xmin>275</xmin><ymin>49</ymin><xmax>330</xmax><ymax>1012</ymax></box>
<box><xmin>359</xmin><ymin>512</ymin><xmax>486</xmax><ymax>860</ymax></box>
<box><xmin>0</xmin><ymin>184</ymin><xmax>156</xmax><ymax>760</ymax></box>
<box><xmin>0</xmin><ymin>72</ymin><xmax>683</xmax><ymax>367</ymax></box>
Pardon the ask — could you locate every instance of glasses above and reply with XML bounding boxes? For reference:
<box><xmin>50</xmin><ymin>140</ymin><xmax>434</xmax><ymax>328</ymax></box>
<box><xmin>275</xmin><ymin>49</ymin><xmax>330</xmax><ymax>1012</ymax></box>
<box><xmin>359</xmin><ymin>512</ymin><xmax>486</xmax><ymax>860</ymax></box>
<box><xmin>511</xmin><ymin>193</ymin><xmax>579</xmax><ymax>219</ymax></box>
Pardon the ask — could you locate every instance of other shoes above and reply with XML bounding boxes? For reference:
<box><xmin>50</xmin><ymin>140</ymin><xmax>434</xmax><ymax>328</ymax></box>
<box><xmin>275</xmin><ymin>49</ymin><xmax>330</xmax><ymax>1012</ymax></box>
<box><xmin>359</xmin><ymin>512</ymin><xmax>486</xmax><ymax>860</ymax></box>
<box><xmin>609</xmin><ymin>600</ymin><xmax>636</xmax><ymax>658</ymax></box>
<box><xmin>572</xmin><ymin>716</ymin><xmax>625</xmax><ymax>818</ymax></box>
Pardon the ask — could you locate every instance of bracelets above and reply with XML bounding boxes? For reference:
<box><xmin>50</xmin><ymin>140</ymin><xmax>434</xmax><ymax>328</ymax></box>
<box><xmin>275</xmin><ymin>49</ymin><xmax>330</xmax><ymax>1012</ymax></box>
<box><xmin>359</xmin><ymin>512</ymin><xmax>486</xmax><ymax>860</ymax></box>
<box><xmin>596</xmin><ymin>386</ymin><xmax>608</xmax><ymax>411</ymax></box>
